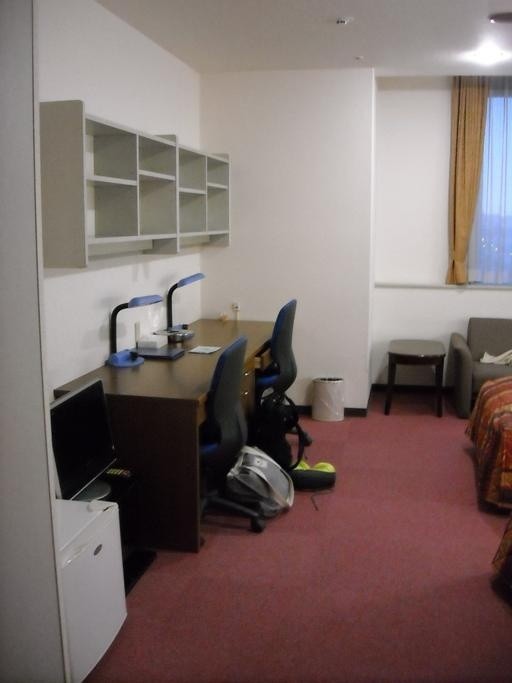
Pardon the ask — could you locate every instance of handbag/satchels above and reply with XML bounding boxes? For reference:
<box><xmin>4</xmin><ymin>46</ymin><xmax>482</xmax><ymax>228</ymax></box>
<box><xmin>218</xmin><ymin>445</ymin><xmax>294</xmax><ymax>517</ymax></box>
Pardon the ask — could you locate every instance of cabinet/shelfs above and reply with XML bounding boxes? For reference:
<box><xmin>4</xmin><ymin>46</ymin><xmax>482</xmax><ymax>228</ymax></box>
<box><xmin>100</xmin><ymin>463</ymin><xmax>158</xmax><ymax>599</ymax></box>
<box><xmin>50</xmin><ymin>497</ymin><xmax>128</xmax><ymax>681</ymax></box>
<box><xmin>39</xmin><ymin>99</ymin><xmax>230</xmax><ymax>267</ymax></box>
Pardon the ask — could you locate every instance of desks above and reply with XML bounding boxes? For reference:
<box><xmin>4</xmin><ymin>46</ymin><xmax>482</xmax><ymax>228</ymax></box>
<box><xmin>384</xmin><ymin>340</ymin><xmax>445</xmax><ymax>418</ymax></box>
<box><xmin>53</xmin><ymin>317</ymin><xmax>281</xmax><ymax>554</ymax></box>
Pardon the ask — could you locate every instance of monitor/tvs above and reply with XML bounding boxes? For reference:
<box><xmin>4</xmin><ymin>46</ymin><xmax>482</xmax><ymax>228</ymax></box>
<box><xmin>50</xmin><ymin>378</ymin><xmax>117</xmax><ymax>502</ymax></box>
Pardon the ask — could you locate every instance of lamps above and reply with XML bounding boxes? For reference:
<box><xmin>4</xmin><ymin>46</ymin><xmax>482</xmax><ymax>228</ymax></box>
<box><xmin>105</xmin><ymin>294</ymin><xmax>163</xmax><ymax>368</ymax></box>
<box><xmin>153</xmin><ymin>272</ymin><xmax>206</xmax><ymax>343</ymax></box>
<box><xmin>488</xmin><ymin>12</ymin><xmax>512</xmax><ymax>27</ymax></box>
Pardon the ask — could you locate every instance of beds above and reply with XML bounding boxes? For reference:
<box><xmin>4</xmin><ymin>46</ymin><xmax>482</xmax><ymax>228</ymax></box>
<box><xmin>466</xmin><ymin>375</ymin><xmax>512</xmax><ymax>512</ymax></box>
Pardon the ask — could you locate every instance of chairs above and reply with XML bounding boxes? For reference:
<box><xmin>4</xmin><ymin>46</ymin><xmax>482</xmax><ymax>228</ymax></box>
<box><xmin>255</xmin><ymin>299</ymin><xmax>313</xmax><ymax>449</ymax></box>
<box><xmin>200</xmin><ymin>336</ymin><xmax>264</xmax><ymax>532</ymax></box>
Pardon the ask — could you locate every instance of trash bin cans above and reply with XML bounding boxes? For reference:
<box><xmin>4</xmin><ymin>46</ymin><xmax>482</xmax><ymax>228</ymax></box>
<box><xmin>312</xmin><ymin>378</ymin><xmax>346</xmax><ymax>422</ymax></box>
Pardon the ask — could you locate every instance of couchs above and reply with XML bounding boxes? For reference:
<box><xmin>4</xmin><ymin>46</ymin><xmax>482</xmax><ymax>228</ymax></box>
<box><xmin>450</xmin><ymin>318</ymin><xmax>511</xmax><ymax>417</ymax></box>
